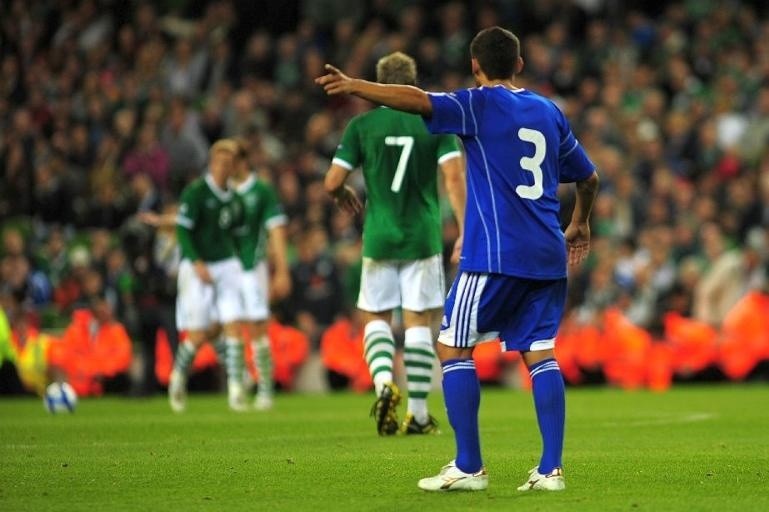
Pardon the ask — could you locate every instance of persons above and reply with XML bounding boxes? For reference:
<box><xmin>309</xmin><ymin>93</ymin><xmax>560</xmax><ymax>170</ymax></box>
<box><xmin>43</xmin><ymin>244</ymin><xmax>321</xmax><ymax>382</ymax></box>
<box><xmin>169</xmin><ymin>138</ymin><xmax>290</xmax><ymax>411</ymax></box>
<box><xmin>0</xmin><ymin>1</ymin><xmax>769</xmax><ymax>394</ymax></box>
<box><xmin>314</xmin><ymin>27</ymin><xmax>601</xmax><ymax>492</ymax></box>
<box><xmin>323</xmin><ymin>52</ymin><xmax>467</xmax><ymax>436</ymax></box>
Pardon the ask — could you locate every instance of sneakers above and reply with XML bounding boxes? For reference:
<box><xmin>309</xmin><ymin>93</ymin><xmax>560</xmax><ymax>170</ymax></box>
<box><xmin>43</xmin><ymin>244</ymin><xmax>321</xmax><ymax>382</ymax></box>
<box><xmin>371</xmin><ymin>383</ymin><xmax>402</xmax><ymax>435</ymax></box>
<box><xmin>229</xmin><ymin>391</ymin><xmax>272</xmax><ymax>410</ymax></box>
<box><xmin>403</xmin><ymin>414</ymin><xmax>441</xmax><ymax>435</ymax></box>
<box><xmin>417</xmin><ymin>459</ymin><xmax>487</xmax><ymax>491</ymax></box>
<box><xmin>169</xmin><ymin>384</ymin><xmax>184</xmax><ymax>410</ymax></box>
<box><xmin>518</xmin><ymin>466</ymin><xmax>564</xmax><ymax>491</ymax></box>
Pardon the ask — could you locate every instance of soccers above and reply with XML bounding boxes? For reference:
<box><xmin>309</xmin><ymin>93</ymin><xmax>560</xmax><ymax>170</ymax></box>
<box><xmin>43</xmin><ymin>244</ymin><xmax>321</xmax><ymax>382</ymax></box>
<box><xmin>45</xmin><ymin>381</ymin><xmax>77</xmax><ymax>413</ymax></box>
